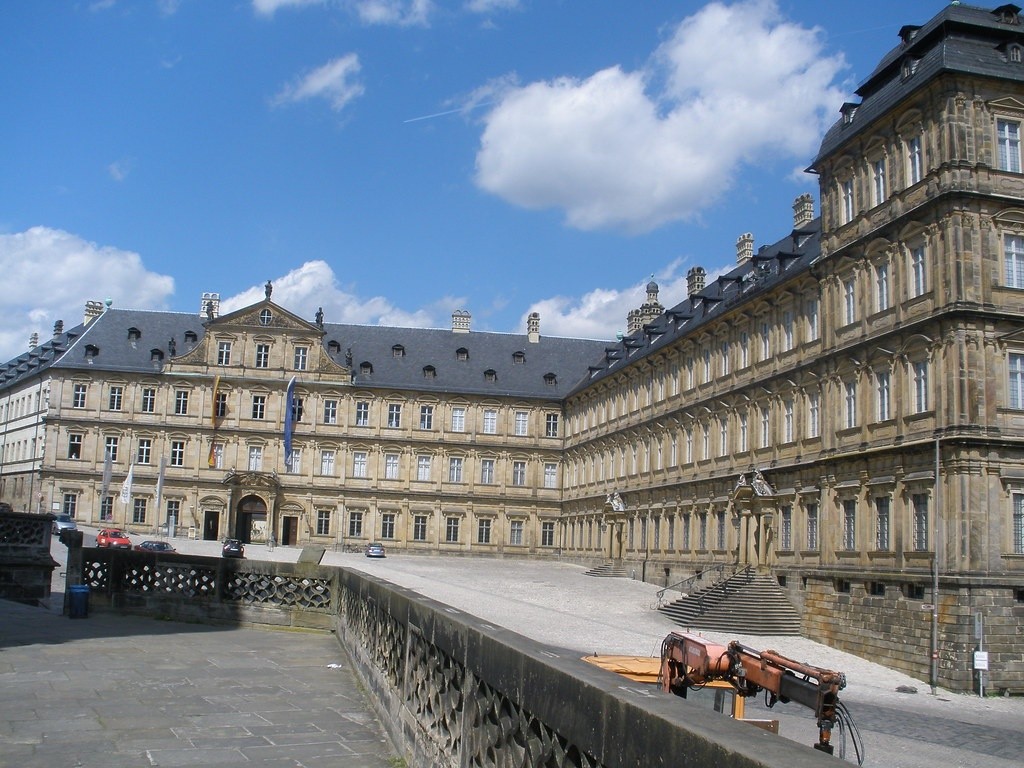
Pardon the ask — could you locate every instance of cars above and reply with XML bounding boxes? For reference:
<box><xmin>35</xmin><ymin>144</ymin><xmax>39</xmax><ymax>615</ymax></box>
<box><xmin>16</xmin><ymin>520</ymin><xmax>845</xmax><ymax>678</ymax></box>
<box><xmin>95</xmin><ymin>528</ymin><xmax>132</xmax><ymax>549</ymax></box>
<box><xmin>134</xmin><ymin>541</ymin><xmax>179</xmax><ymax>555</ymax></box>
<box><xmin>222</xmin><ymin>539</ymin><xmax>245</xmax><ymax>557</ymax></box>
<box><xmin>364</xmin><ymin>543</ymin><xmax>385</xmax><ymax>557</ymax></box>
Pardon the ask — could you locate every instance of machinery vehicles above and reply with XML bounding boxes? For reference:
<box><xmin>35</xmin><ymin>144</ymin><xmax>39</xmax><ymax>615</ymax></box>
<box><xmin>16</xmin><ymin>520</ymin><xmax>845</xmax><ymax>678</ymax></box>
<box><xmin>580</xmin><ymin>630</ymin><xmax>865</xmax><ymax>766</ymax></box>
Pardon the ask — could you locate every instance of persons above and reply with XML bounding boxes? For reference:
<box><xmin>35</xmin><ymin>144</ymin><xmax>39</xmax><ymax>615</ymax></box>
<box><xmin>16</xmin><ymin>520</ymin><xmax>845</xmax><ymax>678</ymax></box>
<box><xmin>315</xmin><ymin>307</ymin><xmax>323</xmax><ymax>328</ymax></box>
<box><xmin>168</xmin><ymin>337</ymin><xmax>176</xmax><ymax>356</ymax></box>
<box><xmin>206</xmin><ymin>302</ymin><xmax>214</xmax><ymax>317</ymax></box>
<box><xmin>345</xmin><ymin>347</ymin><xmax>352</xmax><ymax>366</ymax></box>
<box><xmin>265</xmin><ymin>280</ymin><xmax>272</xmax><ymax>297</ymax></box>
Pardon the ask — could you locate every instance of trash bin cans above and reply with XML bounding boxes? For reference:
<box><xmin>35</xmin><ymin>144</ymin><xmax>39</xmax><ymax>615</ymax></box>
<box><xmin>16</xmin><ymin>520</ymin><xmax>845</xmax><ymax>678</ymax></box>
<box><xmin>69</xmin><ymin>585</ymin><xmax>90</xmax><ymax>618</ymax></box>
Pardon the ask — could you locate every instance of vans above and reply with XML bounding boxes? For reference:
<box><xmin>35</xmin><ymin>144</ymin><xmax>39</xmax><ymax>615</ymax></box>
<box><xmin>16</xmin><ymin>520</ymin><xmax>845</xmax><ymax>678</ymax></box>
<box><xmin>48</xmin><ymin>512</ymin><xmax>79</xmax><ymax>535</ymax></box>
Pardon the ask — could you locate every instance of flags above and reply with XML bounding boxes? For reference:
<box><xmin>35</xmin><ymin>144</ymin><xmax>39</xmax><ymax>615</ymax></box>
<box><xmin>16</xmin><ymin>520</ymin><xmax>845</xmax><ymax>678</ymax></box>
<box><xmin>284</xmin><ymin>379</ymin><xmax>294</xmax><ymax>465</ymax></box>
<box><xmin>119</xmin><ymin>455</ymin><xmax>135</xmax><ymax>503</ymax></box>
<box><xmin>101</xmin><ymin>452</ymin><xmax>112</xmax><ymax>501</ymax></box>
<box><xmin>208</xmin><ymin>377</ymin><xmax>220</xmax><ymax>466</ymax></box>
<box><xmin>155</xmin><ymin>457</ymin><xmax>166</xmax><ymax>507</ymax></box>
